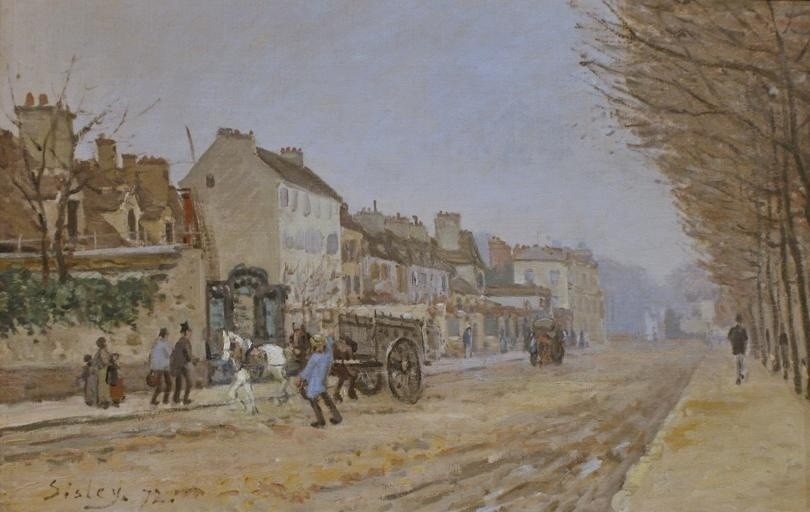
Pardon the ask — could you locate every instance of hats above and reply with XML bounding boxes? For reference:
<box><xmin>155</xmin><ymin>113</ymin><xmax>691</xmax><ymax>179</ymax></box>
<box><xmin>308</xmin><ymin>333</ymin><xmax>328</xmax><ymax>345</ymax></box>
<box><xmin>158</xmin><ymin>327</ymin><xmax>171</xmax><ymax>336</ymax></box>
<box><xmin>178</xmin><ymin>319</ymin><xmax>190</xmax><ymax>333</ymax></box>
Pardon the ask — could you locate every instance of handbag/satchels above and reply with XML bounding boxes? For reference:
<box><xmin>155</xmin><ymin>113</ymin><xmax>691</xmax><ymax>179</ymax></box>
<box><xmin>144</xmin><ymin>370</ymin><xmax>159</xmax><ymax>386</ymax></box>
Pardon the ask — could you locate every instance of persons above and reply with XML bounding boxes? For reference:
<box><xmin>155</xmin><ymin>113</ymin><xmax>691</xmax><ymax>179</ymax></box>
<box><xmin>104</xmin><ymin>352</ymin><xmax>127</xmax><ymax>409</ymax></box>
<box><xmin>93</xmin><ymin>337</ymin><xmax>113</xmax><ymax>411</ymax></box>
<box><xmin>562</xmin><ymin>328</ymin><xmax>570</xmax><ymax>361</ymax></box>
<box><xmin>779</xmin><ymin>322</ymin><xmax>793</xmax><ymax>379</ymax></box>
<box><xmin>726</xmin><ymin>313</ymin><xmax>750</xmax><ymax>386</ymax></box>
<box><xmin>528</xmin><ymin>331</ymin><xmax>539</xmax><ymax>366</ymax></box>
<box><xmin>335</xmin><ymin>334</ymin><xmax>359</xmax><ymax>404</ymax></box>
<box><xmin>462</xmin><ymin>323</ymin><xmax>475</xmax><ymax>360</ymax></box>
<box><xmin>295</xmin><ymin>334</ymin><xmax>343</xmax><ymax>428</ymax></box>
<box><xmin>79</xmin><ymin>353</ymin><xmax>99</xmax><ymax>407</ymax></box>
<box><xmin>576</xmin><ymin>328</ymin><xmax>586</xmax><ymax>352</ymax></box>
<box><xmin>148</xmin><ymin>328</ymin><xmax>174</xmax><ymax>407</ymax></box>
<box><xmin>169</xmin><ymin>321</ymin><xmax>201</xmax><ymax>405</ymax></box>
